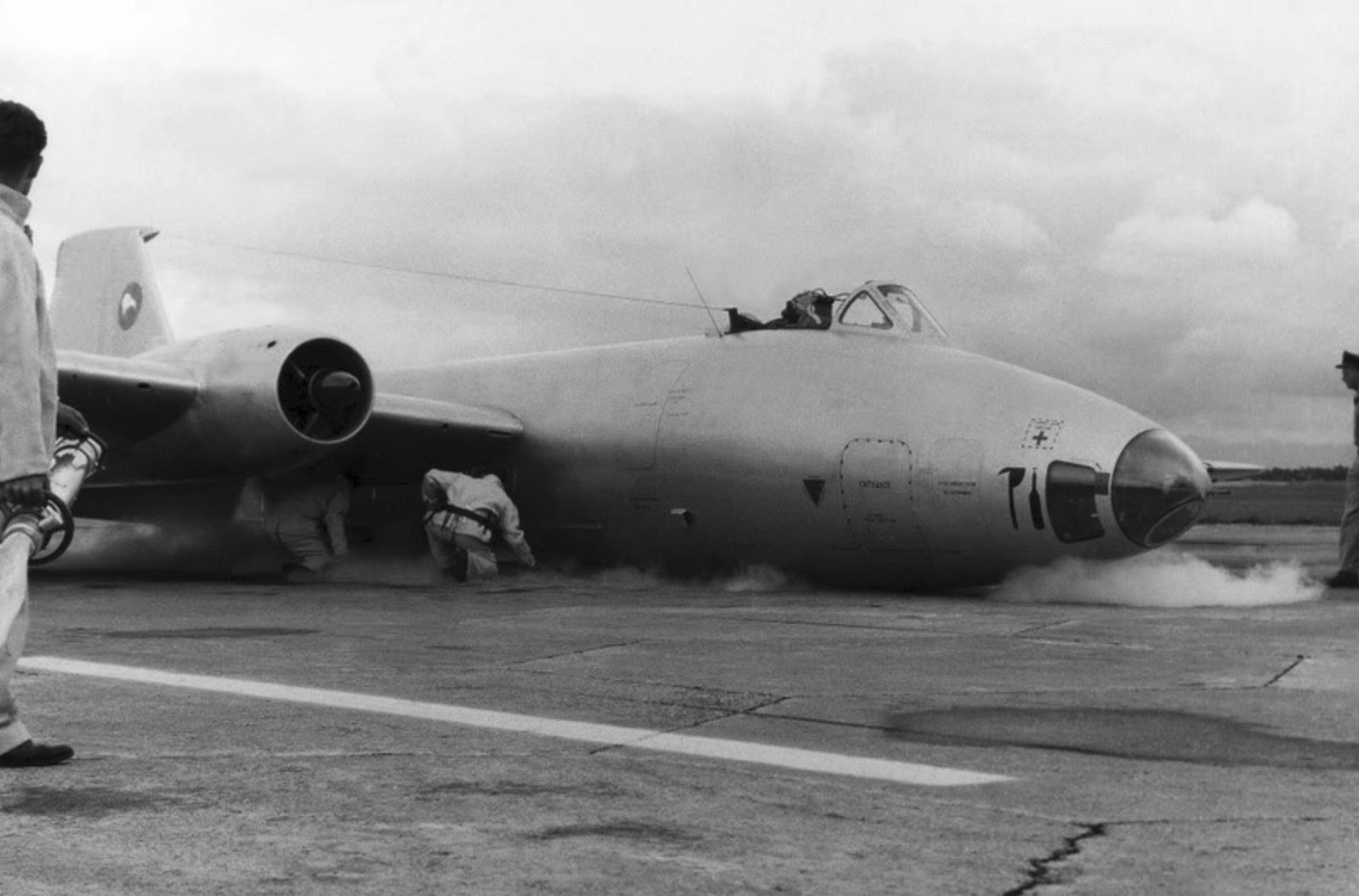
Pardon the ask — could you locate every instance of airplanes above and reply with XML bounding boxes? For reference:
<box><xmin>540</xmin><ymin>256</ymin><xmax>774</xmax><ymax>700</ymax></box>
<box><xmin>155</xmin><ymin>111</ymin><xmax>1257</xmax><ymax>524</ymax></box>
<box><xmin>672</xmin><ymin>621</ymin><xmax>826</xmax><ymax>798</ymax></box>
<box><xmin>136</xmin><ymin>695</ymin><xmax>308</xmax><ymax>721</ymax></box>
<box><xmin>49</xmin><ymin>222</ymin><xmax>1271</xmax><ymax>592</ymax></box>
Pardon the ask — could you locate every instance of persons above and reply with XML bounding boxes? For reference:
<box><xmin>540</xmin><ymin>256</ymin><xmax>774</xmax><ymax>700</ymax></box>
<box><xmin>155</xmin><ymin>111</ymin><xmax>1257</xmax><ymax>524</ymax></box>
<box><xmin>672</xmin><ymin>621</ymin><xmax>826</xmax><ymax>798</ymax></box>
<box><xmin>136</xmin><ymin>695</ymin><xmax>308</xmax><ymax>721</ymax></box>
<box><xmin>0</xmin><ymin>99</ymin><xmax>89</xmax><ymax>768</ymax></box>
<box><xmin>420</xmin><ymin>468</ymin><xmax>541</xmax><ymax>584</ymax></box>
<box><xmin>1322</xmin><ymin>350</ymin><xmax>1359</xmax><ymax>586</ymax></box>
<box><xmin>268</xmin><ymin>469</ymin><xmax>364</xmax><ymax>577</ymax></box>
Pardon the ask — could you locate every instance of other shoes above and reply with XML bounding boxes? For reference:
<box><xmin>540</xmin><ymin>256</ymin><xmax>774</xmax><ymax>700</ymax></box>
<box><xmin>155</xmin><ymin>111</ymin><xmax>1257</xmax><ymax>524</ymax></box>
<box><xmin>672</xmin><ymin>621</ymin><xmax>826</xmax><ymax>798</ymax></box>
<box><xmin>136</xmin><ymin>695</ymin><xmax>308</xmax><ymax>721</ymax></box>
<box><xmin>454</xmin><ymin>549</ymin><xmax>468</xmax><ymax>583</ymax></box>
<box><xmin>290</xmin><ymin>570</ymin><xmax>320</xmax><ymax>585</ymax></box>
<box><xmin>0</xmin><ymin>741</ymin><xmax>74</xmax><ymax>767</ymax></box>
